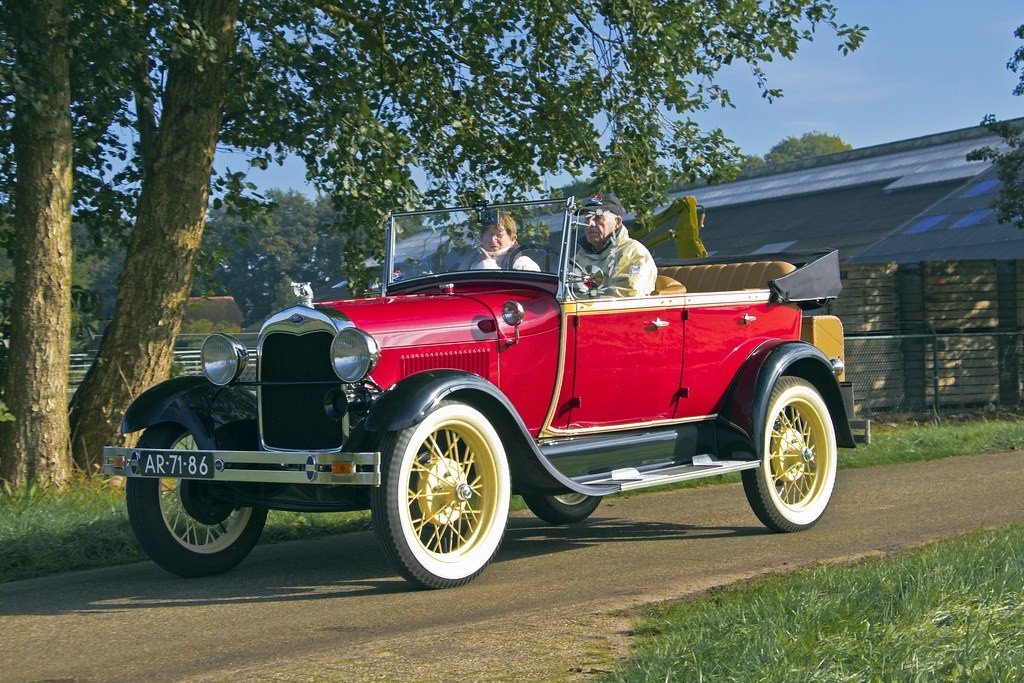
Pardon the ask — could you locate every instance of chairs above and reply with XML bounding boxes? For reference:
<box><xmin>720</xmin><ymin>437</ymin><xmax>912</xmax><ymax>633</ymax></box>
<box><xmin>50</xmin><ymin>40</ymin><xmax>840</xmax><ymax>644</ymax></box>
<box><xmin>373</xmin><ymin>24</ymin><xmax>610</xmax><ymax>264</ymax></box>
<box><xmin>652</xmin><ymin>276</ymin><xmax>685</xmax><ymax>298</ymax></box>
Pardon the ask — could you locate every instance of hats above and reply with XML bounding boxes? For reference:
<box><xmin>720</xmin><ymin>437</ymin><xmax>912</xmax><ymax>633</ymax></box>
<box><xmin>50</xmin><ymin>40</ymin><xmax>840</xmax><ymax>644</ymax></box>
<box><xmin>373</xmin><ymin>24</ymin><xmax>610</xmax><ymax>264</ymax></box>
<box><xmin>573</xmin><ymin>195</ymin><xmax>622</xmax><ymax>217</ymax></box>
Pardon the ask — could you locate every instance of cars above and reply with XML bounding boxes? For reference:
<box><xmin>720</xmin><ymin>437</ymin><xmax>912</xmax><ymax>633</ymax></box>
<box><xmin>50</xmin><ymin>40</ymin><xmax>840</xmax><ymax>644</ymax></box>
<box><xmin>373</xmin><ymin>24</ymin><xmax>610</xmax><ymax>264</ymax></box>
<box><xmin>101</xmin><ymin>198</ymin><xmax>874</xmax><ymax>590</ymax></box>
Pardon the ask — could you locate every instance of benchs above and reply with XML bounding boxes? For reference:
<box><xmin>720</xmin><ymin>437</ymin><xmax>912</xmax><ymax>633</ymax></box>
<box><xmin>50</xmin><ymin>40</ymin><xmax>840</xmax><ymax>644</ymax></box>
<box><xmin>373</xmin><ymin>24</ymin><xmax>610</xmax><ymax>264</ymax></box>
<box><xmin>657</xmin><ymin>261</ymin><xmax>797</xmax><ymax>293</ymax></box>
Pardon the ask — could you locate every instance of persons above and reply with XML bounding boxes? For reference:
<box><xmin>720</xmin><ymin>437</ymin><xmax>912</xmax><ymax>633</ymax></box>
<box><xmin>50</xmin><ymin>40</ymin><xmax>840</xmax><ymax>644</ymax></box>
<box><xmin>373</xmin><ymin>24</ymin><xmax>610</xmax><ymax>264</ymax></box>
<box><xmin>562</xmin><ymin>193</ymin><xmax>658</xmax><ymax>300</ymax></box>
<box><xmin>458</xmin><ymin>212</ymin><xmax>541</xmax><ymax>275</ymax></box>
